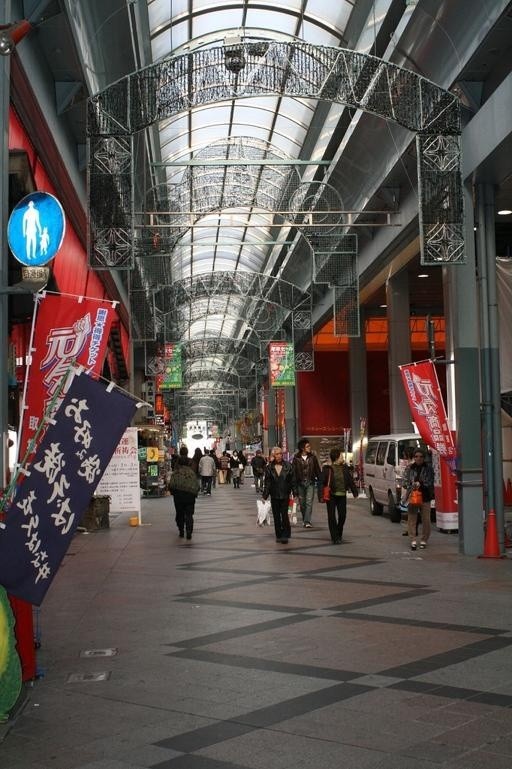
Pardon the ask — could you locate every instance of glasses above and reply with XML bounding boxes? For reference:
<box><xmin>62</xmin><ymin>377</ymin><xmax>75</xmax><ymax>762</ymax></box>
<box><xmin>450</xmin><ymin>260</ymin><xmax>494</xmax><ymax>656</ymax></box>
<box><xmin>415</xmin><ymin>455</ymin><xmax>424</xmax><ymax>458</ymax></box>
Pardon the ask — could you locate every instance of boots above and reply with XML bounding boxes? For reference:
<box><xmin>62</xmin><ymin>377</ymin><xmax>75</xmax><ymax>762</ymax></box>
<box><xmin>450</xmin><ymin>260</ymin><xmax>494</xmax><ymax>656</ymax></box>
<box><xmin>233</xmin><ymin>477</ymin><xmax>240</xmax><ymax>488</ymax></box>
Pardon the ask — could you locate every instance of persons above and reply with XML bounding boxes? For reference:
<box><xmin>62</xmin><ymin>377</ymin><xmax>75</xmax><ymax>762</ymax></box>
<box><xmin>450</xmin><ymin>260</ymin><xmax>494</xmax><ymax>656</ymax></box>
<box><xmin>262</xmin><ymin>447</ymin><xmax>300</xmax><ymax>545</ymax></box>
<box><xmin>317</xmin><ymin>448</ymin><xmax>359</xmax><ymax>545</ymax></box>
<box><xmin>394</xmin><ymin>446</ymin><xmax>420</xmax><ymax>537</ymax></box>
<box><xmin>401</xmin><ymin>449</ymin><xmax>435</xmax><ymax>550</ymax></box>
<box><xmin>171</xmin><ymin>442</ymin><xmax>267</xmax><ymax>494</ymax></box>
<box><xmin>169</xmin><ymin>458</ymin><xmax>199</xmax><ymax>540</ymax></box>
<box><xmin>291</xmin><ymin>439</ymin><xmax>322</xmax><ymax>528</ymax></box>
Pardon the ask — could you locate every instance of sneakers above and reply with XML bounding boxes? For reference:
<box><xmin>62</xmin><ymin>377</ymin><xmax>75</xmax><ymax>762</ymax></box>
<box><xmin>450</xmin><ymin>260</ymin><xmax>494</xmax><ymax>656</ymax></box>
<box><xmin>302</xmin><ymin>521</ymin><xmax>312</xmax><ymax>527</ymax></box>
<box><xmin>334</xmin><ymin>537</ymin><xmax>342</xmax><ymax>544</ymax></box>
<box><xmin>276</xmin><ymin>536</ymin><xmax>288</xmax><ymax>544</ymax></box>
<box><xmin>411</xmin><ymin>541</ymin><xmax>426</xmax><ymax>550</ymax></box>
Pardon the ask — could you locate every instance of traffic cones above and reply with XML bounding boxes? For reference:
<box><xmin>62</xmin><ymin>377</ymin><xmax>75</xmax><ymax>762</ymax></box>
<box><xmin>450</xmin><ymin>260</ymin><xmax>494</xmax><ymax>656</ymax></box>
<box><xmin>505</xmin><ymin>478</ymin><xmax>512</xmax><ymax>507</ymax></box>
<box><xmin>479</xmin><ymin>509</ymin><xmax>503</xmax><ymax>559</ymax></box>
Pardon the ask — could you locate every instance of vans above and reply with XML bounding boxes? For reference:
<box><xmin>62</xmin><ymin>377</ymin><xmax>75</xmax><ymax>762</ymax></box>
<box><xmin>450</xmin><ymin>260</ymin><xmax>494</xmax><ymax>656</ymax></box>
<box><xmin>363</xmin><ymin>432</ymin><xmax>436</xmax><ymax>524</ymax></box>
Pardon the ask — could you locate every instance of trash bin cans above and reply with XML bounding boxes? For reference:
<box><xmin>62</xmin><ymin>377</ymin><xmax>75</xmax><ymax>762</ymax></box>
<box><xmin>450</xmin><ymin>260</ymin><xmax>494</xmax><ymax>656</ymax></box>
<box><xmin>85</xmin><ymin>495</ymin><xmax>111</xmax><ymax>529</ymax></box>
<box><xmin>245</xmin><ymin>460</ymin><xmax>252</xmax><ymax>475</ymax></box>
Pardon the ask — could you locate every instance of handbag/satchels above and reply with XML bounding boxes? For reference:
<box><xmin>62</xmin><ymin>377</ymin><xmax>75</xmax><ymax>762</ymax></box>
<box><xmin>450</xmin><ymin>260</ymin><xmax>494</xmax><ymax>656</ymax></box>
<box><xmin>410</xmin><ymin>488</ymin><xmax>423</xmax><ymax>506</ymax></box>
<box><xmin>256</xmin><ymin>500</ymin><xmax>271</xmax><ymax>527</ymax></box>
<box><xmin>288</xmin><ymin>499</ymin><xmax>298</xmax><ymax>527</ymax></box>
<box><xmin>320</xmin><ymin>487</ymin><xmax>330</xmax><ymax>503</ymax></box>
